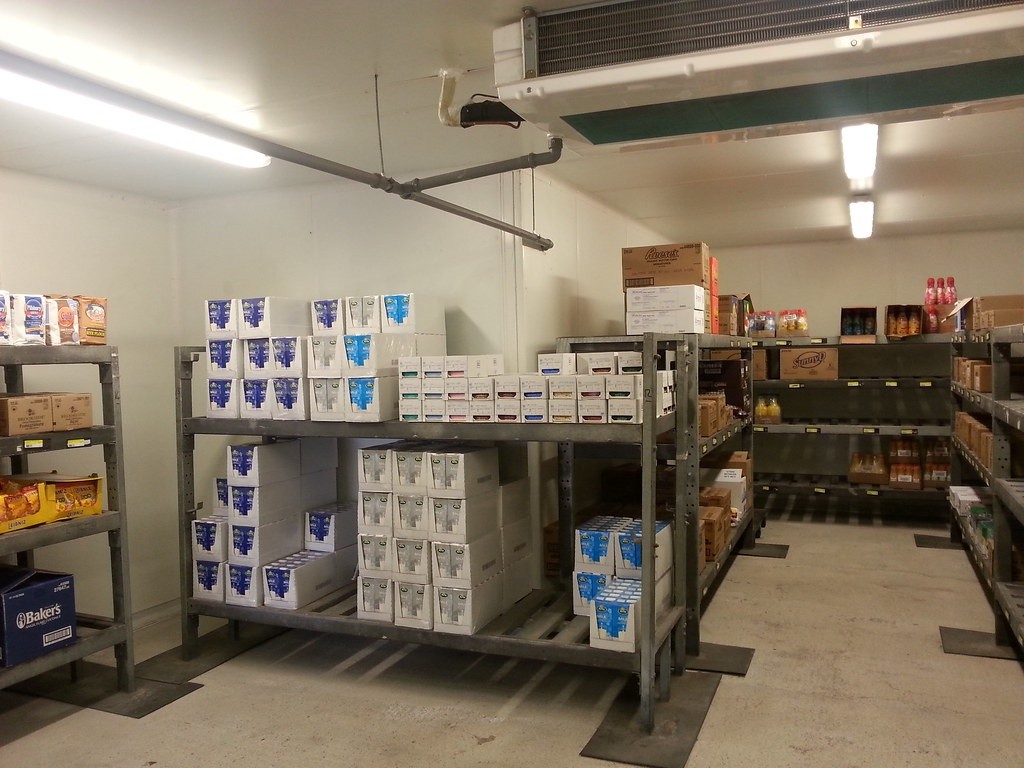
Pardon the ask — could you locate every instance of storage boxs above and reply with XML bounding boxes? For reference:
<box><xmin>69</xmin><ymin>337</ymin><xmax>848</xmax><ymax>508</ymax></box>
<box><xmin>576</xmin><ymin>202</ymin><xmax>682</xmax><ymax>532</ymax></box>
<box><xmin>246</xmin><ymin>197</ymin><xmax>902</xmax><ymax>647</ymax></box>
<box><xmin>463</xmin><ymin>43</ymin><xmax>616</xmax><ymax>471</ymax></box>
<box><xmin>0</xmin><ymin>470</ymin><xmax>103</xmax><ymax>535</ymax></box>
<box><xmin>192</xmin><ymin>241</ymin><xmax>1024</xmax><ymax>654</ymax></box>
<box><xmin>0</xmin><ymin>563</ymin><xmax>76</xmax><ymax>668</ymax></box>
<box><xmin>0</xmin><ymin>391</ymin><xmax>93</xmax><ymax>437</ymax></box>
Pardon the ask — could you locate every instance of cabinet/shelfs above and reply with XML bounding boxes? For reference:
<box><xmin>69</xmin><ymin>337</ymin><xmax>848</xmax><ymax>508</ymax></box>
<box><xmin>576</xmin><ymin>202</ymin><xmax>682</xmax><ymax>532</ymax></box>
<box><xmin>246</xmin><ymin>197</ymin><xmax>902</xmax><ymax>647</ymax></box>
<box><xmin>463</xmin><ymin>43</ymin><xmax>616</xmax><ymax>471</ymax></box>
<box><xmin>177</xmin><ymin>331</ymin><xmax>1023</xmax><ymax>768</ymax></box>
<box><xmin>0</xmin><ymin>345</ymin><xmax>204</xmax><ymax>719</ymax></box>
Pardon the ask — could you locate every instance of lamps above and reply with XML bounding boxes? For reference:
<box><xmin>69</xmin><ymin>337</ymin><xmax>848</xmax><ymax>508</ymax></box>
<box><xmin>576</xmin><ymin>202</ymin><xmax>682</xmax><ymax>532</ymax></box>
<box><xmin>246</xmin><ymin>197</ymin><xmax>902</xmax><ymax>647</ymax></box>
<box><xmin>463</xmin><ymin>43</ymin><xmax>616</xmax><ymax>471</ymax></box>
<box><xmin>849</xmin><ymin>196</ymin><xmax>874</xmax><ymax>239</ymax></box>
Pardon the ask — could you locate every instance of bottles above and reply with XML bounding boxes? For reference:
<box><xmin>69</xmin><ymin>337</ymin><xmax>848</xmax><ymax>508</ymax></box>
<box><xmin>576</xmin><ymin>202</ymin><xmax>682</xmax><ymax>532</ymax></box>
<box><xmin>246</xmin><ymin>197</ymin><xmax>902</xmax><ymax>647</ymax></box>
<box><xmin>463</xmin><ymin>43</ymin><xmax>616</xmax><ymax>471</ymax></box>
<box><xmin>945</xmin><ymin>277</ymin><xmax>957</xmax><ymax>332</ymax></box>
<box><xmin>849</xmin><ymin>436</ymin><xmax>950</xmax><ymax>484</ymax></box>
<box><xmin>936</xmin><ymin>278</ymin><xmax>946</xmax><ymax>334</ymax></box>
<box><xmin>754</xmin><ymin>399</ymin><xmax>767</xmax><ymax>424</ymax></box>
<box><xmin>925</xmin><ymin>278</ymin><xmax>938</xmax><ymax>333</ymax></box>
<box><xmin>767</xmin><ymin>399</ymin><xmax>781</xmax><ymax>425</ymax></box>
<box><xmin>749</xmin><ymin>309</ymin><xmax>807</xmax><ymax>331</ymax></box>
<box><xmin>843</xmin><ymin>312</ymin><xmax>920</xmax><ymax>336</ymax></box>
